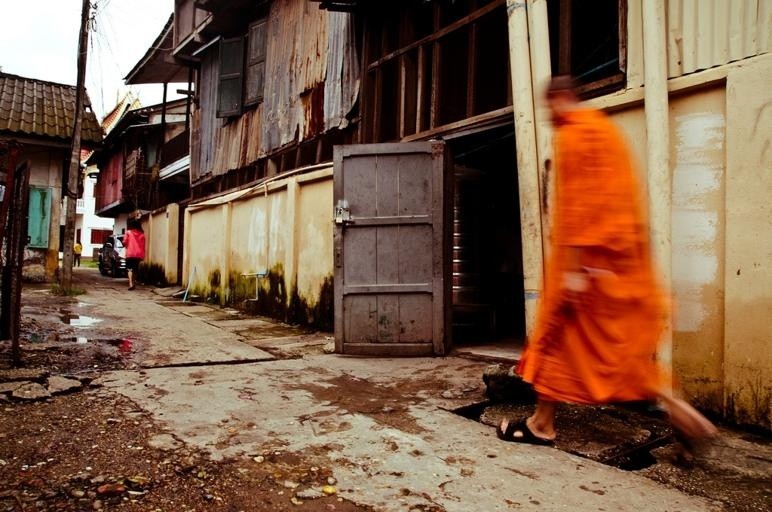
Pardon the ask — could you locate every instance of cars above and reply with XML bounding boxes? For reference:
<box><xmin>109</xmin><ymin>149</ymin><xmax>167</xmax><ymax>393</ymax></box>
<box><xmin>99</xmin><ymin>234</ymin><xmax>134</xmax><ymax>279</ymax></box>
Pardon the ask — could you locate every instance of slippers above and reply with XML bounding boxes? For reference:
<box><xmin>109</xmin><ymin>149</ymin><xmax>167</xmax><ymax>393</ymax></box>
<box><xmin>495</xmin><ymin>418</ymin><xmax>553</xmax><ymax>445</ymax></box>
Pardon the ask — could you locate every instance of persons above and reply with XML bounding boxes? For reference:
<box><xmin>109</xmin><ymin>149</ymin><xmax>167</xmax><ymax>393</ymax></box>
<box><xmin>122</xmin><ymin>220</ymin><xmax>145</xmax><ymax>290</ymax></box>
<box><xmin>500</xmin><ymin>75</ymin><xmax>719</xmax><ymax>461</ymax></box>
<box><xmin>73</xmin><ymin>240</ymin><xmax>82</xmax><ymax>267</ymax></box>
